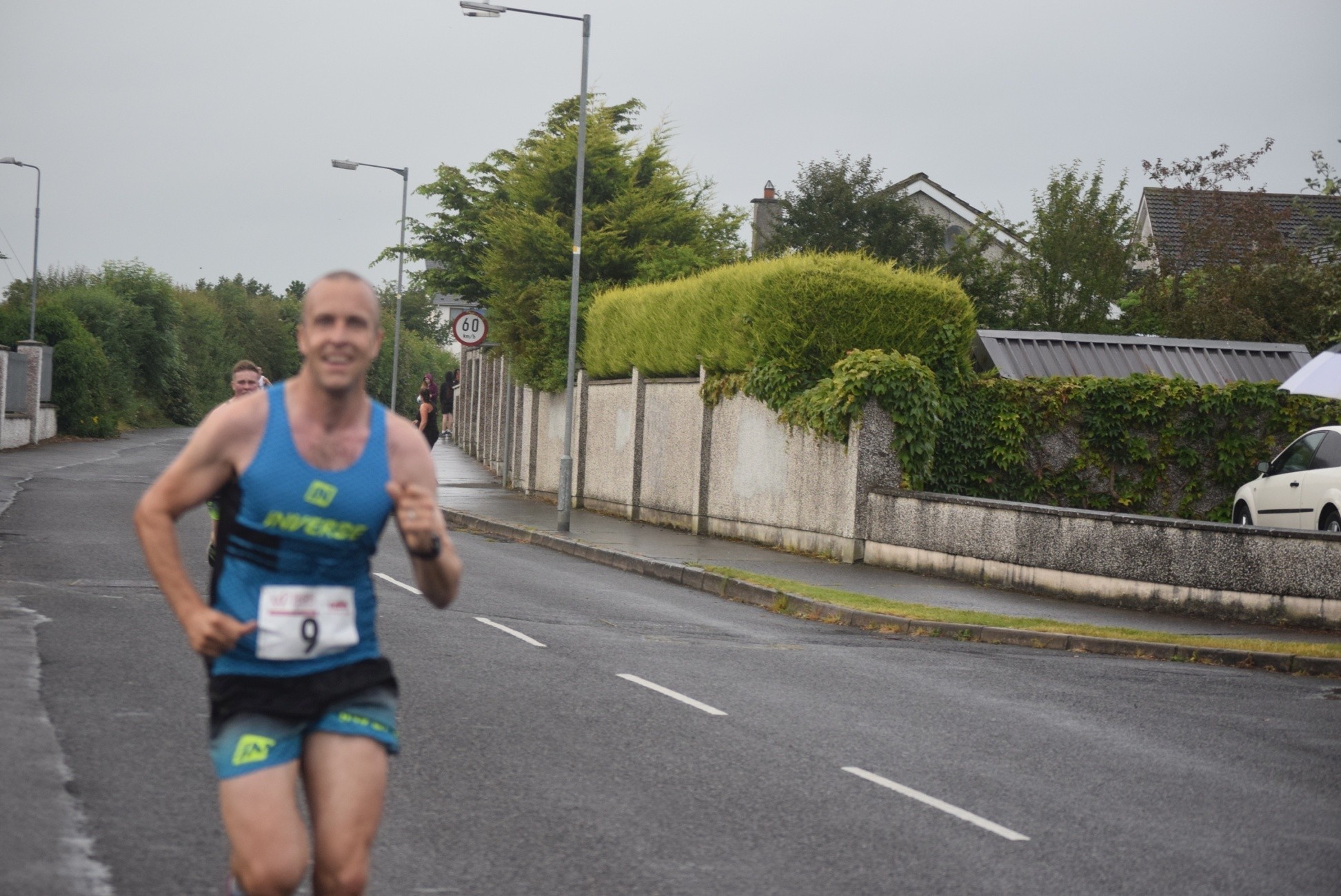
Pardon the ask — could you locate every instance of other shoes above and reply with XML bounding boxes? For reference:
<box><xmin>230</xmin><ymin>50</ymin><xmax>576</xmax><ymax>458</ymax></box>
<box><xmin>440</xmin><ymin>432</ymin><xmax>453</xmax><ymax>437</ymax></box>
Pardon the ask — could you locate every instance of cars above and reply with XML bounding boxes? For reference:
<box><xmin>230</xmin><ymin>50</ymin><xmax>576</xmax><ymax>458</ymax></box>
<box><xmin>1231</xmin><ymin>426</ymin><xmax>1340</xmax><ymax>532</ymax></box>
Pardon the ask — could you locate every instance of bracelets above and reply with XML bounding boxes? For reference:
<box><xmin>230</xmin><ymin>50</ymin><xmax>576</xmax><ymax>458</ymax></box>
<box><xmin>405</xmin><ymin>531</ymin><xmax>441</xmax><ymax>562</ymax></box>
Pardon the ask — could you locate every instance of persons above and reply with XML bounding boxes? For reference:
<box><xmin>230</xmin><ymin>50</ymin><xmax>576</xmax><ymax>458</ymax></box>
<box><xmin>205</xmin><ymin>361</ymin><xmax>262</xmax><ymax>567</ymax></box>
<box><xmin>133</xmin><ymin>270</ymin><xmax>464</xmax><ymax>896</ymax></box>
<box><xmin>412</xmin><ymin>368</ymin><xmax>460</xmax><ymax>455</ymax></box>
<box><xmin>256</xmin><ymin>365</ymin><xmax>274</xmax><ymax>392</ymax></box>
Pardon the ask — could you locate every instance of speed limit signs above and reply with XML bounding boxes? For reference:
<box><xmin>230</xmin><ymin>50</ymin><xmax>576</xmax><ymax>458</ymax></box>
<box><xmin>454</xmin><ymin>311</ymin><xmax>489</xmax><ymax>346</ymax></box>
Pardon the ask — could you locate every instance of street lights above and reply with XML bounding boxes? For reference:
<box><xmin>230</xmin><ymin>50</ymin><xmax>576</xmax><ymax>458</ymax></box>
<box><xmin>458</xmin><ymin>0</ymin><xmax>593</xmax><ymax>534</ymax></box>
<box><xmin>0</xmin><ymin>156</ymin><xmax>41</xmax><ymax>340</ymax></box>
<box><xmin>331</xmin><ymin>157</ymin><xmax>409</xmax><ymax>412</ymax></box>
